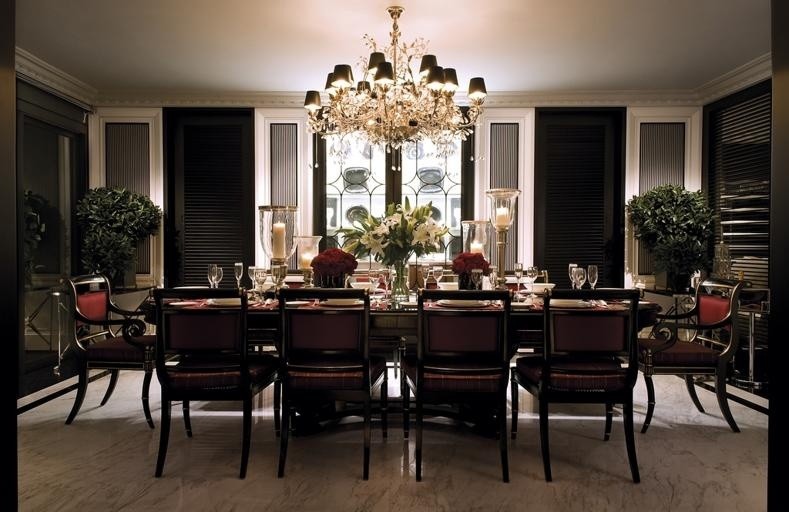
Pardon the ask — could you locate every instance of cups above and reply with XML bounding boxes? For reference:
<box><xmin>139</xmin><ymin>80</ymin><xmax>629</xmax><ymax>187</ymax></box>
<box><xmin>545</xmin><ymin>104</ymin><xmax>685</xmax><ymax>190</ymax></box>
<box><xmin>381</xmin><ymin>269</ymin><xmax>394</xmax><ymax>295</ymax></box>
<box><xmin>254</xmin><ymin>270</ymin><xmax>267</xmax><ymax>298</ymax></box>
<box><xmin>421</xmin><ymin>264</ymin><xmax>429</xmax><ymax>289</ymax></box>
<box><xmin>433</xmin><ymin>266</ymin><xmax>444</xmax><ymax>281</ymax></box>
<box><xmin>248</xmin><ymin>266</ymin><xmax>258</xmax><ymax>288</ymax></box>
<box><xmin>368</xmin><ymin>272</ymin><xmax>380</xmax><ymax>298</ymax></box>
<box><xmin>471</xmin><ymin>269</ymin><xmax>483</xmax><ymax>289</ymax></box>
<box><xmin>527</xmin><ymin>267</ymin><xmax>538</xmax><ymax>289</ymax></box>
<box><xmin>572</xmin><ymin>268</ymin><xmax>586</xmax><ymax>289</ymax></box>
<box><xmin>212</xmin><ymin>266</ymin><xmax>223</xmax><ymax>288</ymax></box>
<box><xmin>234</xmin><ymin>262</ymin><xmax>243</xmax><ymax>288</ymax></box>
<box><xmin>207</xmin><ymin>263</ymin><xmax>217</xmax><ymax>289</ymax></box>
<box><xmin>514</xmin><ymin>263</ymin><xmax>523</xmax><ymax>291</ymax></box>
<box><xmin>588</xmin><ymin>265</ymin><xmax>598</xmax><ymax>290</ymax></box>
<box><xmin>568</xmin><ymin>263</ymin><xmax>578</xmax><ymax>289</ymax></box>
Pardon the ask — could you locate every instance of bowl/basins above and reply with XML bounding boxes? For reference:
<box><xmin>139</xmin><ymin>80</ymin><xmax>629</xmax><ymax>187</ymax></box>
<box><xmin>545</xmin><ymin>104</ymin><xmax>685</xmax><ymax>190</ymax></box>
<box><xmin>524</xmin><ymin>283</ymin><xmax>556</xmax><ymax>293</ymax></box>
<box><xmin>327</xmin><ymin>298</ymin><xmax>359</xmax><ymax>304</ymax></box>
<box><xmin>349</xmin><ymin>282</ymin><xmax>379</xmax><ymax>290</ymax></box>
<box><xmin>213</xmin><ymin>299</ymin><xmax>241</xmax><ymax>304</ymax></box>
<box><xmin>551</xmin><ymin>299</ymin><xmax>589</xmax><ymax>308</ymax></box>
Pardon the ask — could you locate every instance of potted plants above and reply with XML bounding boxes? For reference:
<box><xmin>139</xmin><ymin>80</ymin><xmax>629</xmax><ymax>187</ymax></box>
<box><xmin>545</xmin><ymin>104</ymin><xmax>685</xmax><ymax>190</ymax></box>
<box><xmin>624</xmin><ymin>183</ymin><xmax>721</xmax><ymax>293</ymax></box>
<box><xmin>76</xmin><ymin>186</ymin><xmax>162</xmax><ymax>288</ymax></box>
<box><xmin>24</xmin><ymin>189</ymin><xmax>48</xmax><ymax>284</ymax></box>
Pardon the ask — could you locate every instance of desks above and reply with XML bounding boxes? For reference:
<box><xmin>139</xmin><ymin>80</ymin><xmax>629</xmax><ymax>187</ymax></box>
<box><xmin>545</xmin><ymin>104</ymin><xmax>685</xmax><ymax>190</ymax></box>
<box><xmin>52</xmin><ymin>284</ymin><xmax>157</xmax><ymax>344</ymax></box>
<box><xmin>25</xmin><ymin>284</ymin><xmax>58</xmax><ymax>352</ymax></box>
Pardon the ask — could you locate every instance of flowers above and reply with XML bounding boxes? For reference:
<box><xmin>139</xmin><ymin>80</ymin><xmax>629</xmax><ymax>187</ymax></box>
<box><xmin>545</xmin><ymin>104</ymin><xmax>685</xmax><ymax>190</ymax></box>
<box><xmin>310</xmin><ymin>248</ymin><xmax>359</xmax><ymax>276</ymax></box>
<box><xmin>452</xmin><ymin>252</ymin><xmax>493</xmax><ymax>275</ymax></box>
<box><xmin>336</xmin><ymin>197</ymin><xmax>449</xmax><ymax>290</ymax></box>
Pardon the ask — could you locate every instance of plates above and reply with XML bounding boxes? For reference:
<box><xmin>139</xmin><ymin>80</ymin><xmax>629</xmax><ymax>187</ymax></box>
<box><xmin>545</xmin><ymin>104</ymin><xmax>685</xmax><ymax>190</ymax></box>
<box><xmin>206</xmin><ymin>301</ymin><xmax>256</xmax><ymax>307</ymax></box>
<box><xmin>168</xmin><ymin>301</ymin><xmax>199</xmax><ymax>306</ymax></box>
<box><xmin>435</xmin><ymin>302</ymin><xmax>493</xmax><ymax>307</ymax></box>
<box><xmin>172</xmin><ymin>286</ymin><xmax>207</xmax><ymax>290</ymax></box>
<box><xmin>398</xmin><ymin>301</ymin><xmax>418</xmax><ymax>307</ymax></box>
<box><xmin>620</xmin><ymin>301</ymin><xmax>650</xmax><ymax>305</ymax></box>
<box><xmin>510</xmin><ymin>301</ymin><xmax>535</xmax><ymax>308</ymax></box>
<box><xmin>319</xmin><ymin>301</ymin><xmax>364</xmax><ymax>306</ymax></box>
<box><xmin>285</xmin><ymin>300</ymin><xmax>310</xmax><ymax>307</ymax></box>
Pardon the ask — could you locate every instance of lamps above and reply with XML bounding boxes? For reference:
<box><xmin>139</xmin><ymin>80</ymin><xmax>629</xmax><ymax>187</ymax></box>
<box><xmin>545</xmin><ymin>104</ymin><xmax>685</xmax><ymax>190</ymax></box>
<box><xmin>304</xmin><ymin>5</ymin><xmax>487</xmax><ymax>172</ymax></box>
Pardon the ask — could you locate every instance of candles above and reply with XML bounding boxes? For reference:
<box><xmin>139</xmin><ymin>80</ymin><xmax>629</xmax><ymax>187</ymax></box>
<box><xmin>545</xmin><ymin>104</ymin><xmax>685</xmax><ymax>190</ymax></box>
<box><xmin>272</xmin><ymin>220</ymin><xmax>286</xmax><ymax>258</ymax></box>
<box><xmin>301</xmin><ymin>250</ymin><xmax>314</xmax><ymax>268</ymax></box>
<box><xmin>495</xmin><ymin>204</ymin><xmax>510</xmax><ymax>226</ymax></box>
<box><xmin>469</xmin><ymin>242</ymin><xmax>484</xmax><ymax>257</ymax></box>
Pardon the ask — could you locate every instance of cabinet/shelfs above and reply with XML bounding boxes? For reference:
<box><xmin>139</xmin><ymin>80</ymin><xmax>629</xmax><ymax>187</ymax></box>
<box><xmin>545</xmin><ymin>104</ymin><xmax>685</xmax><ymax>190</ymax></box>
<box><xmin>642</xmin><ymin>288</ymin><xmax>695</xmax><ymax>339</ymax></box>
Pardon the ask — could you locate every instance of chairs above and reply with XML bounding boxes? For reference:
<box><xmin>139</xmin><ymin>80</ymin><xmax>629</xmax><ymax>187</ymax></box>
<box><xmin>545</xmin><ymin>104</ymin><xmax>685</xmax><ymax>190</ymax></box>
<box><xmin>403</xmin><ymin>290</ymin><xmax>511</xmax><ymax>484</ymax></box>
<box><xmin>152</xmin><ymin>289</ymin><xmax>281</xmax><ymax>479</ymax></box>
<box><xmin>510</xmin><ymin>288</ymin><xmax>640</xmax><ymax>484</ymax></box>
<box><xmin>279</xmin><ymin>288</ymin><xmax>389</xmax><ymax>481</ymax></box>
<box><xmin>65</xmin><ymin>273</ymin><xmax>156</xmax><ymax>429</ymax></box>
<box><xmin>614</xmin><ymin>277</ymin><xmax>745</xmax><ymax>433</ymax></box>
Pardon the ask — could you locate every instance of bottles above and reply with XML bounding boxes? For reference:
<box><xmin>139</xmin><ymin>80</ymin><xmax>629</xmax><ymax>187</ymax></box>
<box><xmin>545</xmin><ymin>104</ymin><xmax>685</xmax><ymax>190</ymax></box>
<box><xmin>426</xmin><ymin>271</ymin><xmax>437</xmax><ymax>289</ymax></box>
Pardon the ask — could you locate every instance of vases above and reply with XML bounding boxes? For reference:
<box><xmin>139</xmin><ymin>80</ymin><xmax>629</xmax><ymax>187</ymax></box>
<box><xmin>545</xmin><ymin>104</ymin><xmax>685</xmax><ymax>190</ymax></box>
<box><xmin>458</xmin><ymin>273</ymin><xmax>483</xmax><ymax>290</ymax></box>
<box><xmin>321</xmin><ymin>273</ymin><xmax>345</xmax><ymax>288</ymax></box>
<box><xmin>391</xmin><ymin>260</ymin><xmax>410</xmax><ymax>301</ymax></box>
